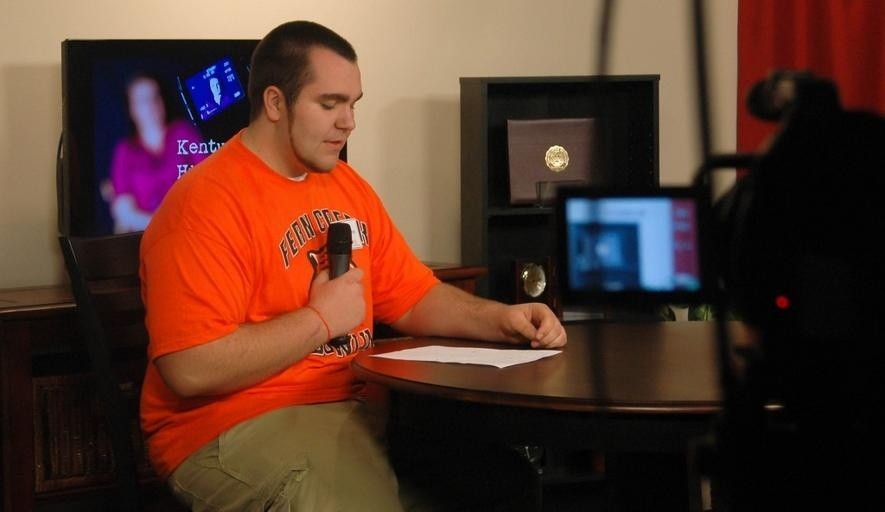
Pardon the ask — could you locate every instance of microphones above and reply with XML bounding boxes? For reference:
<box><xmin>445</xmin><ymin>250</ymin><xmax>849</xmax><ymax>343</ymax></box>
<box><xmin>327</xmin><ymin>221</ymin><xmax>353</xmax><ymax>353</ymax></box>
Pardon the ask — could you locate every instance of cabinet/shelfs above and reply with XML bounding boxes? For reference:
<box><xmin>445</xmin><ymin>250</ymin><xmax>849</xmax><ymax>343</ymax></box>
<box><xmin>0</xmin><ymin>262</ymin><xmax>492</xmax><ymax>512</ymax></box>
<box><xmin>460</xmin><ymin>74</ymin><xmax>659</xmax><ymax>325</ymax></box>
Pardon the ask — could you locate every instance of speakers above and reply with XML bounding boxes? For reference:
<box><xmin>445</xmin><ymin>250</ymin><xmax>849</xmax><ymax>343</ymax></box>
<box><xmin>484</xmin><ymin>204</ymin><xmax>560</xmax><ymax>316</ymax></box>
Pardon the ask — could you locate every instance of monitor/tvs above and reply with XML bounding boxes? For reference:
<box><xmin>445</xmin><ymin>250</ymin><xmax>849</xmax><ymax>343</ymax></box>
<box><xmin>58</xmin><ymin>36</ymin><xmax>348</xmax><ymax>315</ymax></box>
<box><xmin>560</xmin><ymin>186</ymin><xmax>708</xmax><ymax>320</ymax></box>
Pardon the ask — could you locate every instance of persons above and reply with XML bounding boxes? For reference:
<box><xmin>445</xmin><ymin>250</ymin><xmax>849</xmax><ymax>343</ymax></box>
<box><xmin>99</xmin><ymin>73</ymin><xmax>210</xmax><ymax>236</ymax></box>
<box><xmin>137</xmin><ymin>21</ymin><xmax>568</xmax><ymax>512</ymax></box>
<box><xmin>202</xmin><ymin>75</ymin><xmax>234</xmax><ymax>118</ymax></box>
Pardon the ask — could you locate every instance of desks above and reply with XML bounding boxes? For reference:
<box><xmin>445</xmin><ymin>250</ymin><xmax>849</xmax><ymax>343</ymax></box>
<box><xmin>351</xmin><ymin>321</ymin><xmax>787</xmax><ymax>512</ymax></box>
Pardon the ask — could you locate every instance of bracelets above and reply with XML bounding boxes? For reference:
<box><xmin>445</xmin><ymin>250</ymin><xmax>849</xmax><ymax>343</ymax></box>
<box><xmin>305</xmin><ymin>304</ymin><xmax>331</xmax><ymax>343</ymax></box>
